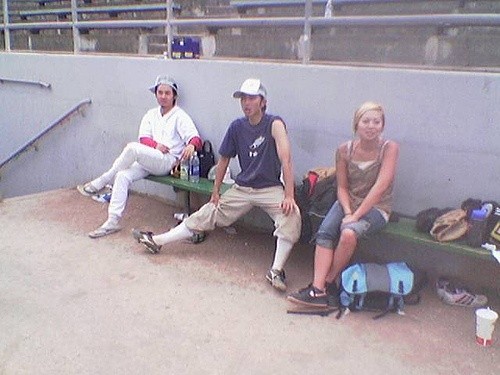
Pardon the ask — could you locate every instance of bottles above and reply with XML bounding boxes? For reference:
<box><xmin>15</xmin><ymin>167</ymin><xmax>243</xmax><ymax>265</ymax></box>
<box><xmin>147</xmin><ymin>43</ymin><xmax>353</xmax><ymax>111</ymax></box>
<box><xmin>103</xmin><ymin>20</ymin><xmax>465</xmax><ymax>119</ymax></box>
<box><xmin>190</xmin><ymin>152</ymin><xmax>200</xmax><ymax>183</ymax></box>
<box><xmin>180</xmin><ymin>158</ymin><xmax>188</xmax><ymax>181</ymax></box>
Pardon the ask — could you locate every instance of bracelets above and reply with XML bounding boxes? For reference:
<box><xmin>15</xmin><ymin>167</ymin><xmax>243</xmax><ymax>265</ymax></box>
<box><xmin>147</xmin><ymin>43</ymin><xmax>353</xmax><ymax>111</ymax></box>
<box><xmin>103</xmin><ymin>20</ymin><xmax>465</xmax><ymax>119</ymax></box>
<box><xmin>346</xmin><ymin>213</ymin><xmax>352</xmax><ymax>215</ymax></box>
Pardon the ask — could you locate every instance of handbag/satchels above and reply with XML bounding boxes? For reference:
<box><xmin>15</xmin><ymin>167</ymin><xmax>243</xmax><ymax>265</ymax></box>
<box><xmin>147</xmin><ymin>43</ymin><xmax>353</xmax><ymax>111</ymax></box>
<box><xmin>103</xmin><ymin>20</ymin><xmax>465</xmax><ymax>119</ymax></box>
<box><xmin>336</xmin><ymin>262</ymin><xmax>414</xmax><ymax>320</ymax></box>
<box><xmin>430</xmin><ymin>209</ymin><xmax>468</xmax><ymax>242</ymax></box>
<box><xmin>197</xmin><ymin>140</ymin><xmax>214</xmax><ymax>177</ymax></box>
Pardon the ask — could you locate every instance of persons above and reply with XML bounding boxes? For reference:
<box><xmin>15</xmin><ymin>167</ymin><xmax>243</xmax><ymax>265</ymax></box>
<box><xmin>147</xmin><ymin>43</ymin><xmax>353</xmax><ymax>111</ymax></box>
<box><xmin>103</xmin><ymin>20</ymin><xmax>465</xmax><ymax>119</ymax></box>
<box><xmin>77</xmin><ymin>76</ymin><xmax>202</xmax><ymax>239</ymax></box>
<box><xmin>130</xmin><ymin>78</ymin><xmax>302</xmax><ymax>291</ymax></box>
<box><xmin>287</xmin><ymin>101</ymin><xmax>399</xmax><ymax>315</ymax></box>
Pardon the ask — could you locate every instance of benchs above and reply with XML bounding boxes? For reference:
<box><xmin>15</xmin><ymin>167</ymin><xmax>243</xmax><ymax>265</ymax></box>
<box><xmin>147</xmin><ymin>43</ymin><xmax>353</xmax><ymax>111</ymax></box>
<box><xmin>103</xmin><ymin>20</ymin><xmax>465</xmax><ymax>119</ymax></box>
<box><xmin>142</xmin><ymin>168</ymin><xmax>499</xmax><ymax>268</ymax></box>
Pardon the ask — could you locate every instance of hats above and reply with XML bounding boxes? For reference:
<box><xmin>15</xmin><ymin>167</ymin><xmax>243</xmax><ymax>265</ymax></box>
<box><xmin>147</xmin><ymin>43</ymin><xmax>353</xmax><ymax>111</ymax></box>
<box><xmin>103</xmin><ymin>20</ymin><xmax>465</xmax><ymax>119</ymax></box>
<box><xmin>233</xmin><ymin>79</ymin><xmax>267</xmax><ymax>98</ymax></box>
<box><xmin>149</xmin><ymin>75</ymin><xmax>179</xmax><ymax>94</ymax></box>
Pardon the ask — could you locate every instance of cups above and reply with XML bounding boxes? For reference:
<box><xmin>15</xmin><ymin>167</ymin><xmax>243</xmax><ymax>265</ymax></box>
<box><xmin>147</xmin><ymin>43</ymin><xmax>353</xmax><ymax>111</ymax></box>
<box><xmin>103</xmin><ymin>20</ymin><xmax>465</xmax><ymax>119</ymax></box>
<box><xmin>469</xmin><ymin>210</ymin><xmax>486</xmax><ymax>247</ymax></box>
<box><xmin>474</xmin><ymin>309</ymin><xmax>499</xmax><ymax>347</ymax></box>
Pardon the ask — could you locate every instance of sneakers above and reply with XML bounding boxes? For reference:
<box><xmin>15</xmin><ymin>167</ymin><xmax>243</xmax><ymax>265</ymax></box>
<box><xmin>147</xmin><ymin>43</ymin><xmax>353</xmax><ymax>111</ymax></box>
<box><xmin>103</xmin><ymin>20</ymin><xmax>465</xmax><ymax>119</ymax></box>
<box><xmin>265</xmin><ymin>268</ymin><xmax>286</xmax><ymax>291</ymax></box>
<box><xmin>288</xmin><ymin>285</ymin><xmax>328</xmax><ymax>306</ymax></box>
<box><xmin>443</xmin><ymin>289</ymin><xmax>488</xmax><ymax>307</ymax></box>
<box><xmin>131</xmin><ymin>228</ymin><xmax>162</xmax><ymax>254</ymax></box>
<box><xmin>436</xmin><ymin>276</ymin><xmax>451</xmax><ymax>298</ymax></box>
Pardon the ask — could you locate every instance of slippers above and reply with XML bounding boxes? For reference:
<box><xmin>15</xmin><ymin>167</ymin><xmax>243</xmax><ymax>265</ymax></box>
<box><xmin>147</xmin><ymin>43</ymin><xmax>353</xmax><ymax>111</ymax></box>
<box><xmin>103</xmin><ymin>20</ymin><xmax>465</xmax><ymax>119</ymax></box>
<box><xmin>90</xmin><ymin>227</ymin><xmax>118</xmax><ymax>237</ymax></box>
<box><xmin>77</xmin><ymin>182</ymin><xmax>98</xmax><ymax>196</ymax></box>
